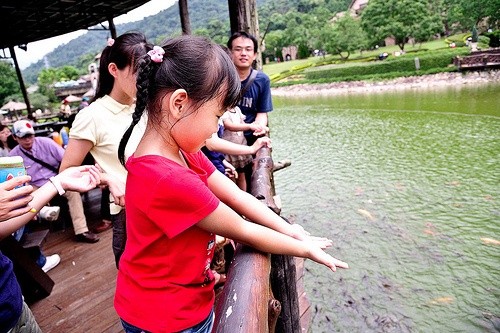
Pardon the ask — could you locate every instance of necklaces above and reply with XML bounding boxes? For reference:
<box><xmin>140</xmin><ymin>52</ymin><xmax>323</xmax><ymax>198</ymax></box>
<box><xmin>229</xmin><ymin>108</ymin><xmax>243</xmax><ymax>137</ymax></box>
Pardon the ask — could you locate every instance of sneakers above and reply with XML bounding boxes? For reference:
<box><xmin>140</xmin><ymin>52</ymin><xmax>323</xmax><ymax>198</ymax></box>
<box><xmin>42</xmin><ymin>254</ymin><xmax>60</xmax><ymax>273</ymax></box>
<box><xmin>39</xmin><ymin>205</ymin><xmax>60</xmax><ymax>221</ymax></box>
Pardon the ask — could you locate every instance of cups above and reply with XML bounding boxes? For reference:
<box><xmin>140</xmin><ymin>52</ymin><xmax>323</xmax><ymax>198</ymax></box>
<box><xmin>0</xmin><ymin>156</ymin><xmax>25</xmax><ymax>209</ymax></box>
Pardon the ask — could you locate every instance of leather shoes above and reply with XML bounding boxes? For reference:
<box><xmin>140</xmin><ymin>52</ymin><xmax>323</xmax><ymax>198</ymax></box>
<box><xmin>75</xmin><ymin>231</ymin><xmax>100</xmax><ymax>243</ymax></box>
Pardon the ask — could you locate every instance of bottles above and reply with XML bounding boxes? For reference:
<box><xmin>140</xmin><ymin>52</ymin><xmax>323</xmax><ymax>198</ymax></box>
<box><xmin>53</xmin><ymin>129</ymin><xmax>68</xmax><ymax>148</ymax></box>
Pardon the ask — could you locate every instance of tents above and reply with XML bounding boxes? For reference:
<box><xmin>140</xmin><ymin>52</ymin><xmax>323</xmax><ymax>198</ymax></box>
<box><xmin>0</xmin><ymin>0</ymin><xmax>152</xmax><ymax>119</ymax></box>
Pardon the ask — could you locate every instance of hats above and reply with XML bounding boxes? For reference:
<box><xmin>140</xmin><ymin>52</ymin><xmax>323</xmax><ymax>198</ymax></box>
<box><xmin>12</xmin><ymin>120</ymin><xmax>35</xmax><ymax>138</ymax></box>
<box><xmin>64</xmin><ymin>99</ymin><xmax>71</xmax><ymax>103</ymax></box>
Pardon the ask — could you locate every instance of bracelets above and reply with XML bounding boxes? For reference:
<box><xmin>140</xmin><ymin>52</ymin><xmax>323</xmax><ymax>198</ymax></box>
<box><xmin>49</xmin><ymin>176</ymin><xmax>65</xmax><ymax>196</ymax></box>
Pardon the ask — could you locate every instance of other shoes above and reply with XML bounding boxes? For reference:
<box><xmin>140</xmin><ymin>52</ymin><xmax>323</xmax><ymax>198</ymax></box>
<box><xmin>93</xmin><ymin>219</ymin><xmax>113</xmax><ymax>233</ymax></box>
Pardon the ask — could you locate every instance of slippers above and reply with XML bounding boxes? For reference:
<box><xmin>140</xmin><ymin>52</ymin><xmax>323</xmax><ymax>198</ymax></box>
<box><xmin>214</xmin><ymin>274</ymin><xmax>227</xmax><ymax>290</ymax></box>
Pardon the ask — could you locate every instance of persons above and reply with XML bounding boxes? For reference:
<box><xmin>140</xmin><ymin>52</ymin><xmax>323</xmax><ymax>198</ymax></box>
<box><xmin>222</xmin><ymin>106</ymin><xmax>270</xmax><ymax>192</ymax></box>
<box><xmin>227</xmin><ymin>32</ymin><xmax>273</xmax><ymax>194</ymax></box>
<box><xmin>0</xmin><ymin>163</ymin><xmax>103</xmax><ymax>333</ymax></box>
<box><xmin>60</xmin><ymin>100</ymin><xmax>71</xmax><ymax>118</ymax></box>
<box><xmin>200</xmin><ymin>118</ymin><xmax>272</xmax><ymax>291</ymax></box>
<box><xmin>59</xmin><ymin>30</ymin><xmax>154</xmax><ymax>270</ymax></box>
<box><xmin>81</xmin><ymin>98</ymin><xmax>89</xmax><ymax>107</ymax></box>
<box><xmin>469</xmin><ymin>38</ymin><xmax>478</xmax><ymax>52</ymax></box>
<box><xmin>0</xmin><ymin>120</ymin><xmax>113</xmax><ymax>243</ymax></box>
<box><xmin>113</xmin><ymin>27</ymin><xmax>349</xmax><ymax>333</ymax></box>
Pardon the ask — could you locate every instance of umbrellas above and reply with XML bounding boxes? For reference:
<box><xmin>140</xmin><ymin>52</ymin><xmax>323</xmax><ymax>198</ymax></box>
<box><xmin>0</xmin><ymin>99</ymin><xmax>34</xmax><ymax>110</ymax></box>
<box><xmin>61</xmin><ymin>94</ymin><xmax>82</xmax><ymax>103</ymax></box>
